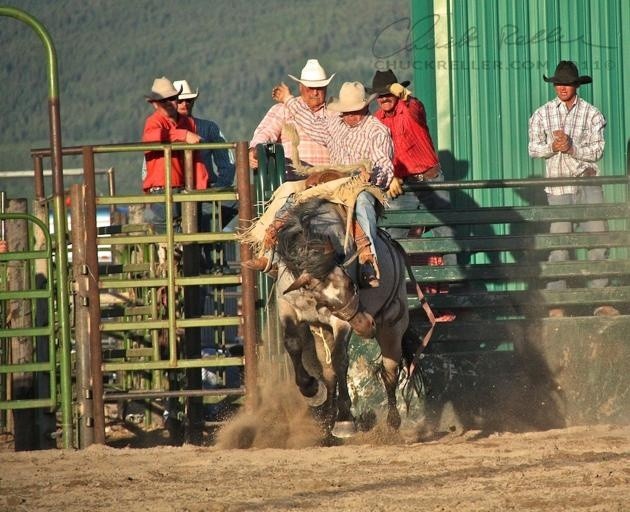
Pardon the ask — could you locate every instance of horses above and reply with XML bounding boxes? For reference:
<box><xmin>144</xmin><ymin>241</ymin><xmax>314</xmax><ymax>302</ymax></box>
<box><xmin>267</xmin><ymin>193</ymin><xmax>438</xmax><ymax>438</ymax></box>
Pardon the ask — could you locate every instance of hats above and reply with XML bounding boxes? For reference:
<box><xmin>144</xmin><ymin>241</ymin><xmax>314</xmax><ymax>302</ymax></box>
<box><xmin>542</xmin><ymin>59</ymin><xmax>594</xmax><ymax>87</ymax></box>
<box><xmin>324</xmin><ymin>80</ymin><xmax>380</xmax><ymax>113</ymax></box>
<box><xmin>288</xmin><ymin>58</ymin><xmax>338</xmax><ymax>89</ymax></box>
<box><xmin>173</xmin><ymin>78</ymin><xmax>200</xmax><ymax>101</ymax></box>
<box><xmin>142</xmin><ymin>75</ymin><xmax>183</xmax><ymax>103</ymax></box>
<box><xmin>363</xmin><ymin>68</ymin><xmax>412</xmax><ymax>97</ymax></box>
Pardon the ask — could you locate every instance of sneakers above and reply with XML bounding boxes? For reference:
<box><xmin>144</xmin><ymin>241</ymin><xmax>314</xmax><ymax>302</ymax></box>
<box><xmin>360</xmin><ymin>263</ymin><xmax>381</xmax><ymax>288</ymax></box>
<box><xmin>592</xmin><ymin>304</ymin><xmax>620</xmax><ymax>318</ymax></box>
<box><xmin>239</xmin><ymin>253</ymin><xmax>277</xmax><ymax>282</ymax></box>
<box><xmin>548</xmin><ymin>305</ymin><xmax>569</xmax><ymax>317</ymax></box>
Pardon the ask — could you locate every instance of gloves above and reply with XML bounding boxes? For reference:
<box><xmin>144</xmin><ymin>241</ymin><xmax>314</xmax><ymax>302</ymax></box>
<box><xmin>388</xmin><ymin>83</ymin><xmax>412</xmax><ymax>103</ymax></box>
<box><xmin>385</xmin><ymin>177</ymin><xmax>404</xmax><ymax>200</ymax></box>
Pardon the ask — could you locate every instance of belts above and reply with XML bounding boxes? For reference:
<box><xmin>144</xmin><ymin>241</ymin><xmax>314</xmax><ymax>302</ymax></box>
<box><xmin>402</xmin><ymin>163</ymin><xmax>441</xmax><ymax>184</ymax></box>
<box><xmin>146</xmin><ymin>181</ymin><xmax>182</xmax><ymax>194</ymax></box>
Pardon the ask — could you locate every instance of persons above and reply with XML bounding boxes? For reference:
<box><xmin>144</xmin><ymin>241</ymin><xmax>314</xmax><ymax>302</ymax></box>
<box><xmin>523</xmin><ymin>61</ymin><xmax>623</xmax><ymax>320</ymax></box>
<box><xmin>365</xmin><ymin>69</ymin><xmax>483</xmax><ymax>325</ymax></box>
<box><xmin>135</xmin><ymin>76</ymin><xmax>242</xmax><ymax>279</ymax></box>
<box><xmin>246</xmin><ymin>58</ymin><xmax>345</xmax><ymax>184</ymax></box>
<box><xmin>240</xmin><ymin>79</ymin><xmax>397</xmax><ymax>288</ymax></box>
